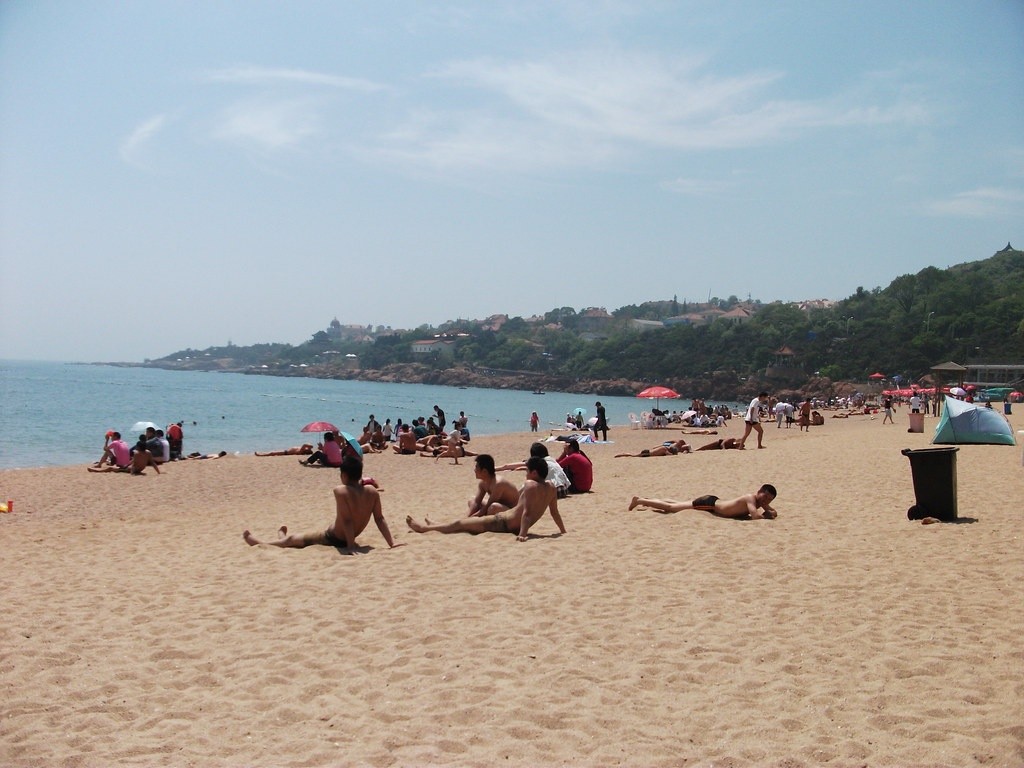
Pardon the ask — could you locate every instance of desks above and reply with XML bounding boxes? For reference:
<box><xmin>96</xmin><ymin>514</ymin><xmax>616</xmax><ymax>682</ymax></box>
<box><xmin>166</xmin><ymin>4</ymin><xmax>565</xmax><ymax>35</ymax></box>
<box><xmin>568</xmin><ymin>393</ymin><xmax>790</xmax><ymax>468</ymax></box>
<box><xmin>654</xmin><ymin>416</ymin><xmax>667</xmax><ymax>427</ymax></box>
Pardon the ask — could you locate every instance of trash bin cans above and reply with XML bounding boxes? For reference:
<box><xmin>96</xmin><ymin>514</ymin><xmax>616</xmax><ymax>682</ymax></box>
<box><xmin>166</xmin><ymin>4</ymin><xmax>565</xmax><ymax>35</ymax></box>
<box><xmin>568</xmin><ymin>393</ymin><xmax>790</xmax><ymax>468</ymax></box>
<box><xmin>1011</xmin><ymin>396</ymin><xmax>1017</xmax><ymax>403</ymax></box>
<box><xmin>1004</xmin><ymin>404</ymin><xmax>1012</xmax><ymax>414</ymax></box>
<box><xmin>902</xmin><ymin>447</ymin><xmax>959</xmax><ymax>521</ymax></box>
<box><xmin>907</xmin><ymin>413</ymin><xmax>925</xmax><ymax>434</ymax></box>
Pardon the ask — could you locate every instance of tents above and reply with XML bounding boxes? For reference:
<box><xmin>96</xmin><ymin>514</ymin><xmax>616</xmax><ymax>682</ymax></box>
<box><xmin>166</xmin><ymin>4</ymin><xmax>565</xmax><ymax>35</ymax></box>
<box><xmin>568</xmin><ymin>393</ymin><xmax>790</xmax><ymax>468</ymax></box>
<box><xmin>929</xmin><ymin>361</ymin><xmax>966</xmax><ymax>383</ymax></box>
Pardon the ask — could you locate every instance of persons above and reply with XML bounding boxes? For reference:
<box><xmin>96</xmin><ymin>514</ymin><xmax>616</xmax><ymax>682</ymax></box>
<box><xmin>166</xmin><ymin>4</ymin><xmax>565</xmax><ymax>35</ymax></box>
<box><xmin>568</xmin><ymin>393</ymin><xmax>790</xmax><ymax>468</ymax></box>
<box><xmin>739</xmin><ymin>392</ymin><xmax>767</xmax><ymax>450</ymax></box>
<box><xmin>254</xmin><ymin>401</ymin><xmax>611</xmax><ymax>547</ymax></box>
<box><xmin>242</xmin><ymin>456</ymin><xmax>408</xmax><ymax>548</ymax></box>
<box><xmin>628</xmin><ymin>483</ymin><xmax>779</xmax><ymax>520</ymax></box>
<box><xmin>86</xmin><ymin>423</ymin><xmax>227</xmax><ymax>476</ymax></box>
<box><xmin>614</xmin><ymin>439</ymin><xmax>691</xmax><ymax>458</ymax></box>
<box><xmin>694</xmin><ymin>438</ymin><xmax>746</xmax><ymax>451</ymax></box>
<box><xmin>663</xmin><ymin>374</ymin><xmax>1019</xmax><ymax>435</ymax></box>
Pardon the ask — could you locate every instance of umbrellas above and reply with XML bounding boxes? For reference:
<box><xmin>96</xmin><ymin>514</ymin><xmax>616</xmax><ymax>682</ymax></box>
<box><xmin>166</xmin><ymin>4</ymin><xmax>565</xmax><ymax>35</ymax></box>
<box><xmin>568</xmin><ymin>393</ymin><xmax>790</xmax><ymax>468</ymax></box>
<box><xmin>131</xmin><ymin>421</ymin><xmax>163</xmax><ymax>431</ymax></box>
<box><xmin>300</xmin><ymin>421</ymin><xmax>339</xmax><ymax>432</ymax></box>
<box><xmin>636</xmin><ymin>384</ymin><xmax>681</xmax><ymax>410</ymax></box>
<box><xmin>868</xmin><ymin>372</ymin><xmax>886</xmax><ymax>379</ymax></box>
<box><xmin>340</xmin><ymin>432</ymin><xmax>363</xmax><ymax>460</ymax></box>
<box><xmin>883</xmin><ymin>384</ymin><xmax>977</xmax><ymax>399</ymax></box>
<box><xmin>1009</xmin><ymin>389</ymin><xmax>1023</xmax><ymax>397</ymax></box>
<box><xmin>572</xmin><ymin>407</ymin><xmax>586</xmax><ymax>415</ymax></box>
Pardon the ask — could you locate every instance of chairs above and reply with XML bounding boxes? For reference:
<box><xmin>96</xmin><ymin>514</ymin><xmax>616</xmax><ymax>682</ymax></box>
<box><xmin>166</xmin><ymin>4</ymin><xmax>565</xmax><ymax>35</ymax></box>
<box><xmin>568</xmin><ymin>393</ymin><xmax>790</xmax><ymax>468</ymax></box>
<box><xmin>629</xmin><ymin>411</ymin><xmax>655</xmax><ymax>430</ymax></box>
